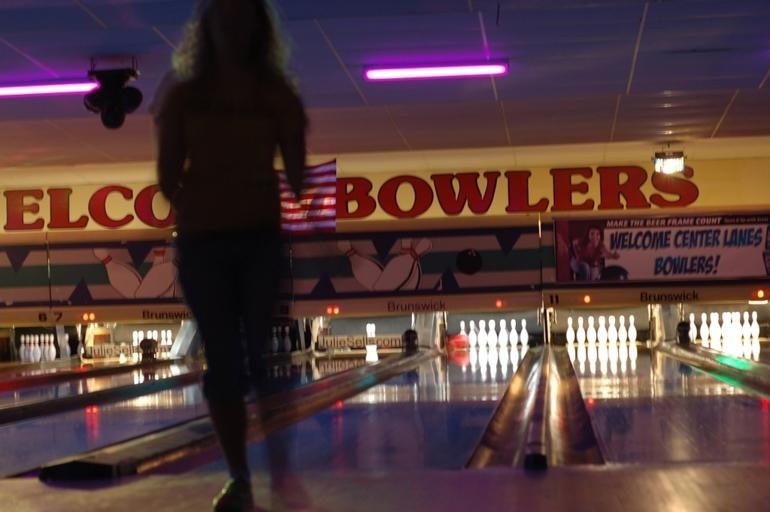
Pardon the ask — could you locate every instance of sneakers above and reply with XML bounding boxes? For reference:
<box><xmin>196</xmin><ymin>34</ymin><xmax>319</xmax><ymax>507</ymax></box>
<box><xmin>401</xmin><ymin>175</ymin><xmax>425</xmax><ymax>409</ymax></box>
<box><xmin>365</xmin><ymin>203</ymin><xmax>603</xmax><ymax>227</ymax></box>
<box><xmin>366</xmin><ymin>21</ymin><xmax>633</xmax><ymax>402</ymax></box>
<box><xmin>211</xmin><ymin>476</ymin><xmax>255</xmax><ymax>512</ymax></box>
<box><xmin>259</xmin><ymin>427</ymin><xmax>293</xmax><ymax>468</ymax></box>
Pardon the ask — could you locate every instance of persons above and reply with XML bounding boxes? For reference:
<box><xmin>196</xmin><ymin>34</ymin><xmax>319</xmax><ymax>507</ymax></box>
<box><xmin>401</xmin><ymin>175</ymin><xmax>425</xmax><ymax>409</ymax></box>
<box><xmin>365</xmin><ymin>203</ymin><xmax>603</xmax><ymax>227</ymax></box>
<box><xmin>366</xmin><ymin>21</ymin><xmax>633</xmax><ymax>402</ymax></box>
<box><xmin>148</xmin><ymin>0</ymin><xmax>312</xmax><ymax>512</ymax></box>
